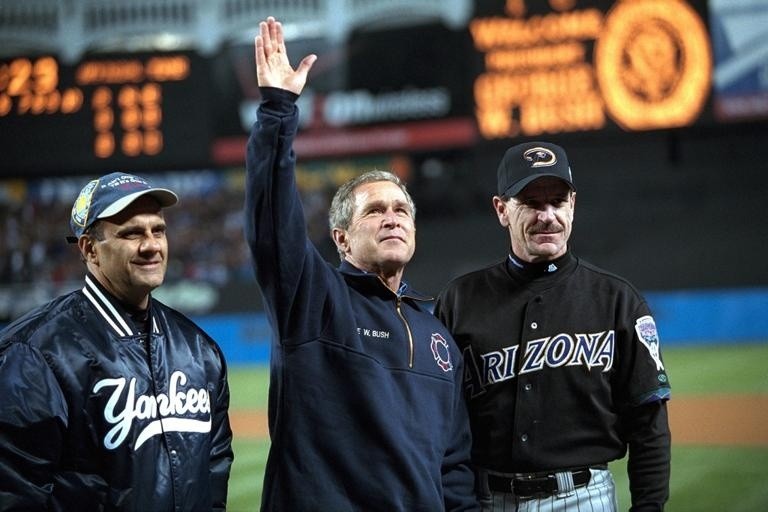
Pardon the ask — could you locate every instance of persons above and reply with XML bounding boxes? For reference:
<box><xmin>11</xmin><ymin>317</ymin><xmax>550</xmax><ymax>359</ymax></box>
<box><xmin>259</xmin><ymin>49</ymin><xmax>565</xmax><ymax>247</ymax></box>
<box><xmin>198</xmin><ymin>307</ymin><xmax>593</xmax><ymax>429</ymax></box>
<box><xmin>1</xmin><ymin>170</ymin><xmax>233</xmax><ymax>512</ymax></box>
<box><xmin>434</xmin><ymin>141</ymin><xmax>670</xmax><ymax>511</ymax></box>
<box><xmin>245</xmin><ymin>17</ymin><xmax>481</xmax><ymax>510</ymax></box>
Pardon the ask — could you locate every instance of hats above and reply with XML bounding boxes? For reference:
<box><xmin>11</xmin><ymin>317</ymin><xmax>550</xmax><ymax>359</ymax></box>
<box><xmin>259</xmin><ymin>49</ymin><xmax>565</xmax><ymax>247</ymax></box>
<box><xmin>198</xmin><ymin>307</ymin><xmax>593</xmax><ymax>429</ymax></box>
<box><xmin>65</xmin><ymin>172</ymin><xmax>179</xmax><ymax>245</ymax></box>
<box><xmin>497</xmin><ymin>142</ymin><xmax>576</xmax><ymax>200</ymax></box>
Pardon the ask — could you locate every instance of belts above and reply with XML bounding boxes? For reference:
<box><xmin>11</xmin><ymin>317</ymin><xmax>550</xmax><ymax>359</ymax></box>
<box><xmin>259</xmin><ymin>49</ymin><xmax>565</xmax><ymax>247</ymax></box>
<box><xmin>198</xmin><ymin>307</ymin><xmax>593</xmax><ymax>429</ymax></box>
<box><xmin>488</xmin><ymin>472</ymin><xmax>590</xmax><ymax>500</ymax></box>
